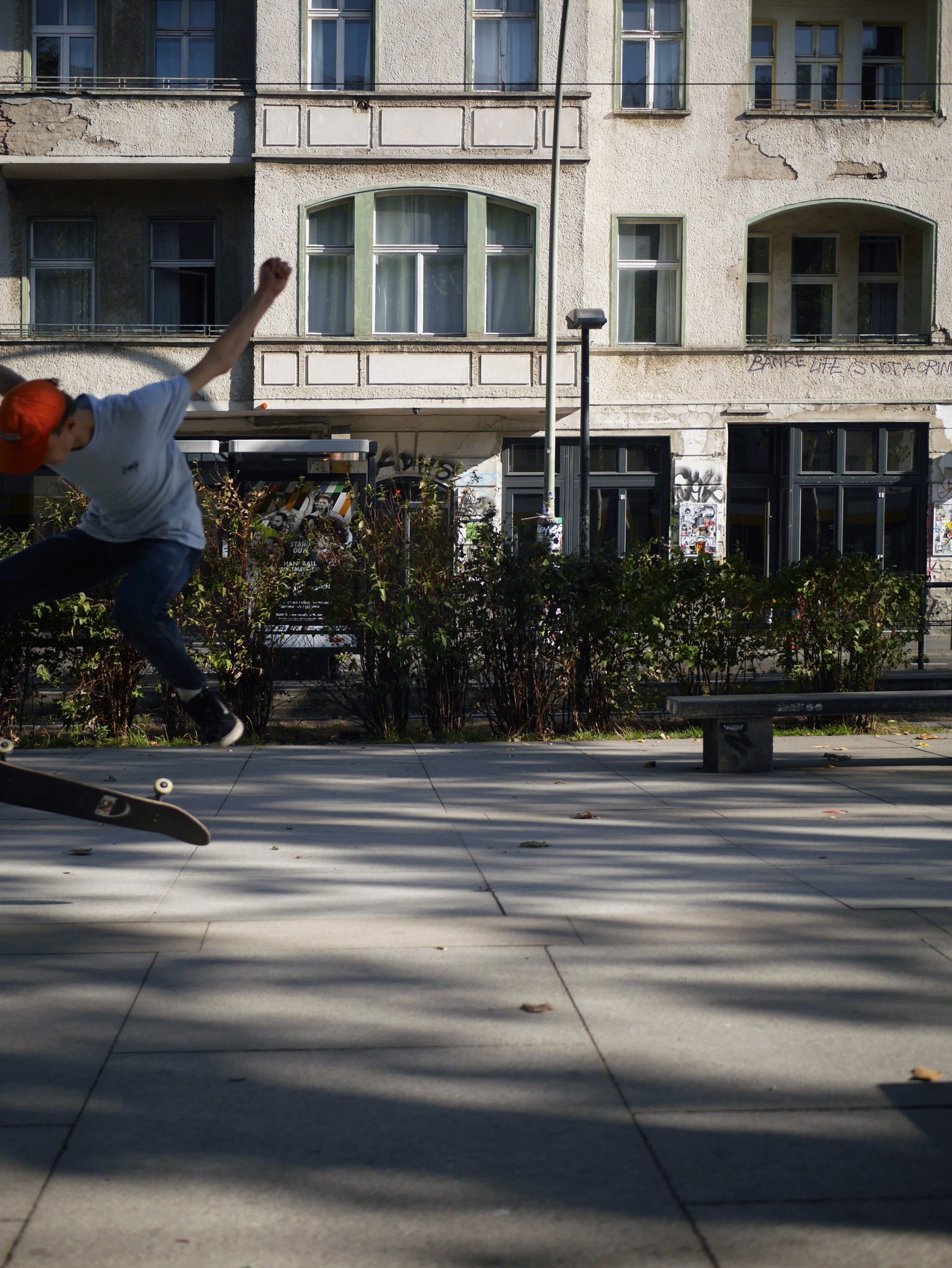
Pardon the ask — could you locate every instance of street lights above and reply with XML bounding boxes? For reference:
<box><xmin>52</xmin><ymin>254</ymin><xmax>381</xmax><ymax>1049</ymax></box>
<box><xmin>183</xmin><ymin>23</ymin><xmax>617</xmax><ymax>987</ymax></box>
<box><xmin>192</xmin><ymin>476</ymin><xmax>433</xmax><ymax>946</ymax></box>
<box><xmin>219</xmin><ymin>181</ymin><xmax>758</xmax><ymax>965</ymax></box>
<box><xmin>565</xmin><ymin>307</ymin><xmax>609</xmax><ymax>726</ymax></box>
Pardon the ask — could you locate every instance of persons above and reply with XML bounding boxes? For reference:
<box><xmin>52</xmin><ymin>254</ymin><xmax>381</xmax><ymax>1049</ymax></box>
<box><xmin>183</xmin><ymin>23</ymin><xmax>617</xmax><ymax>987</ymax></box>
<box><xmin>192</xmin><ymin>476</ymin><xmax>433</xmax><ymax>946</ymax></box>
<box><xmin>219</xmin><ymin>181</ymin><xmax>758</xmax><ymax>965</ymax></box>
<box><xmin>253</xmin><ymin>494</ymin><xmax>353</xmax><ymax>571</ymax></box>
<box><xmin>0</xmin><ymin>256</ymin><xmax>293</xmax><ymax>748</ymax></box>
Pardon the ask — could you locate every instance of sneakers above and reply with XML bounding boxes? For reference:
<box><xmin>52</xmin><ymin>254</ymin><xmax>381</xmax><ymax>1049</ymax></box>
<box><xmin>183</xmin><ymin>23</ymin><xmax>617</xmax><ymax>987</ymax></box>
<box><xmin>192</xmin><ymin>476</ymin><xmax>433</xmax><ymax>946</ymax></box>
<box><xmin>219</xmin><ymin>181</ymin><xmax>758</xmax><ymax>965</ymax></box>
<box><xmin>186</xmin><ymin>692</ymin><xmax>244</xmax><ymax>748</ymax></box>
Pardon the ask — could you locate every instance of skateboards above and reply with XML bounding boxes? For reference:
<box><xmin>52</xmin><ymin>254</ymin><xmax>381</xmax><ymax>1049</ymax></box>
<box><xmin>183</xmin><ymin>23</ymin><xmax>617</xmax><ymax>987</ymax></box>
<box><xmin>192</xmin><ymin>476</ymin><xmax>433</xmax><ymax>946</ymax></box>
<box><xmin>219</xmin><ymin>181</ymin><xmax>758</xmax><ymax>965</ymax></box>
<box><xmin>0</xmin><ymin>734</ymin><xmax>212</xmax><ymax>847</ymax></box>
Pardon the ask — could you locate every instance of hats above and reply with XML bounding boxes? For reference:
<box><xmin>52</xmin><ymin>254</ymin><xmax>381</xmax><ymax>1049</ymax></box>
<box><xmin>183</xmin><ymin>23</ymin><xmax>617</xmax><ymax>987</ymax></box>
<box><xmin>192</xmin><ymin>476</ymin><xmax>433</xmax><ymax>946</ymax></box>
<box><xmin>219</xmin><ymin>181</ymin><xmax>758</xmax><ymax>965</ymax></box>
<box><xmin>0</xmin><ymin>379</ymin><xmax>65</xmax><ymax>475</ymax></box>
